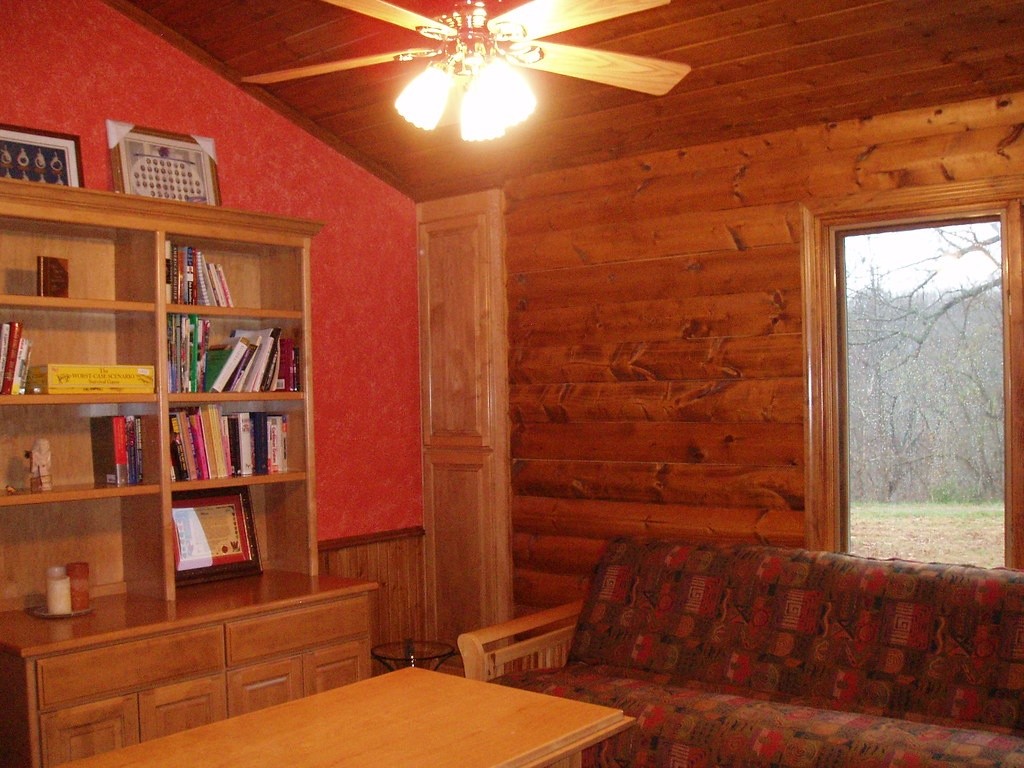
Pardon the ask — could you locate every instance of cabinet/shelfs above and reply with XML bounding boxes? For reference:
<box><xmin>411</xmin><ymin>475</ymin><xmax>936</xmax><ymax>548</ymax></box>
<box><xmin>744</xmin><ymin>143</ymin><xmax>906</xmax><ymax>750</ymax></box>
<box><xmin>0</xmin><ymin>180</ymin><xmax>379</xmax><ymax>768</ymax></box>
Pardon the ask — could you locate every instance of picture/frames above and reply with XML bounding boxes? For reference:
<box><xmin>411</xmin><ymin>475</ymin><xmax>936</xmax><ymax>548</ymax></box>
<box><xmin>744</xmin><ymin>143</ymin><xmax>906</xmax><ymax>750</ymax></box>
<box><xmin>106</xmin><ymin>119</ymin><xmax>222</xmax><ymax>207</ymax></box>
<box><xmin>0</xmin><ymin>123</ymin><xmax>85</xmax><ymax>188</ymax></box>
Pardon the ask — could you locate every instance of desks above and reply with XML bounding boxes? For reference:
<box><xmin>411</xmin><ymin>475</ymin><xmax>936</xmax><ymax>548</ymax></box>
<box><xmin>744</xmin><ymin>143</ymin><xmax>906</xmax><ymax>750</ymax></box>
<box><xmin>370</xmin><ymin>639</ymin><xmax>459</xmax><ymax>673</ymax></box>
<box><xmin>52</xmin><ymin>667</ymin><xmax>633</xmax><ymax>768</ymax></box>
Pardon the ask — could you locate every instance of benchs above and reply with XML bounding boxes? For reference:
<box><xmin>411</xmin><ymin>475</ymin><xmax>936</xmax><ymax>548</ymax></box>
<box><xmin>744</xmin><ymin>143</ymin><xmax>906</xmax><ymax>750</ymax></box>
<box><xmin>457</xmin><ymin>535</ymin><xmax>1024</xmax><ymax>768</ymax></box>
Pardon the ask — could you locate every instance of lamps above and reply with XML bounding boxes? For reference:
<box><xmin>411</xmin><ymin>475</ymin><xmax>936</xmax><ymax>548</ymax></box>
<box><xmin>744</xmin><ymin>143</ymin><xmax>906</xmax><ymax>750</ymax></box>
<box><xmin>394</xmin><ymin>48</ymin><xmax>537</xmax><ymax>142</ymax></box>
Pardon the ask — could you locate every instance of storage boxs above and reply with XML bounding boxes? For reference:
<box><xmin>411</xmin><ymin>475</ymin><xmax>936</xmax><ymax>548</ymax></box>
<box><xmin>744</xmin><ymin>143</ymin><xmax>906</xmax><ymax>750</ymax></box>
<box><xmin>24</xmin><ymin>359</ymin><xmax>156</xmax><ymax>397</ymax></box>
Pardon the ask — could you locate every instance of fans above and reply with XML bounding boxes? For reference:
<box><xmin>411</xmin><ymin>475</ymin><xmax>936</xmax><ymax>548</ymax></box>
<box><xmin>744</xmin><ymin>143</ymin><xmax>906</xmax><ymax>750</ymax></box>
<box><xmin>240</xmin><ymin>0</ymin><xmax>690</xmax><ymax>95</ymax></box>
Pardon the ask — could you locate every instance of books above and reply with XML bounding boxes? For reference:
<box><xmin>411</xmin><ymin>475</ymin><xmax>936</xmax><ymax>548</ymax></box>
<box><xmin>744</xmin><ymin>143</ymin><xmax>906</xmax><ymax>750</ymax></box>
<box><xmin>169</xmin><ymin>404</ymin><xmax>289</xmax><ymax>483</ymax></box>
<box><xmin>165</xmin><ymin>239</ymin><xmax>233</xmax><ymax>308</ymax></box>
<box><xmin>90</xmin><ymin>417</ymin><xmax>143</xmax><ymax>486</ymax></box>
<box><xmin>167</xmin><ymin>313</ymin><xmax>300</xmax><ymax>392</ymax></box>
<box><xmin>0</xmin><ymin>320</ymin><xmax>31</xmax><ymax>394</ymax></box>
<box><xmin>37</xmin><ymin>256</ymin><xmax>69</xmax><ymax>297</ymax></box>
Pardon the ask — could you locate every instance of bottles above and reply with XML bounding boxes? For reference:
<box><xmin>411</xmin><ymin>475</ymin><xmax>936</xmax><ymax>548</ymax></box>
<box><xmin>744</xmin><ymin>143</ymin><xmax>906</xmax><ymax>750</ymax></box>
<box><xmin>45</xmin><ymin>561</ymin><xmax>91</xmax><ymax>615</ymax></box>
